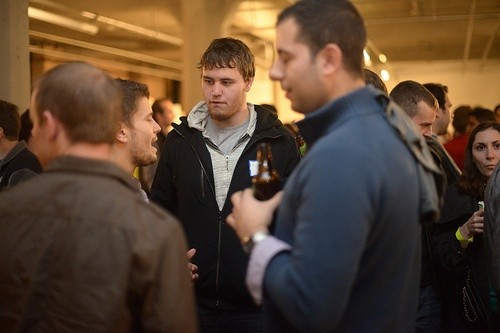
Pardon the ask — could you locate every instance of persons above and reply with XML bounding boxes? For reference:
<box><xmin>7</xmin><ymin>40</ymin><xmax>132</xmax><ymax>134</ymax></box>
<box><xmin>0</xmin><ymin>38</ymin><xmax>305</xmax><ymax>333</ymax></box>
<box><xmin>362</xmin><ymin>68</ymin><xmax>500</xmax><ymax>333</ymax></box>
<box><xmin>226</xmin><ymin>0</ymin><xmax>421</xmax><ymax>333</ymax></box>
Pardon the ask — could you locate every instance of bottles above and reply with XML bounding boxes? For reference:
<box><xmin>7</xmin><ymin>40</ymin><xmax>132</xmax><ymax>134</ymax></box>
<box><xmin>252</xmin><ymin>142</ymin><xmax>280</xmax><ymax>234</ymax></box>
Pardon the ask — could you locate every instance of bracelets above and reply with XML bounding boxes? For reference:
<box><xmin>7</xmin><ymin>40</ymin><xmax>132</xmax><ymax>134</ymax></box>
<box><xmin>242</xmin><ymin>233</ymin><xmax>269</xmax><ymax>254</ymax></box>
